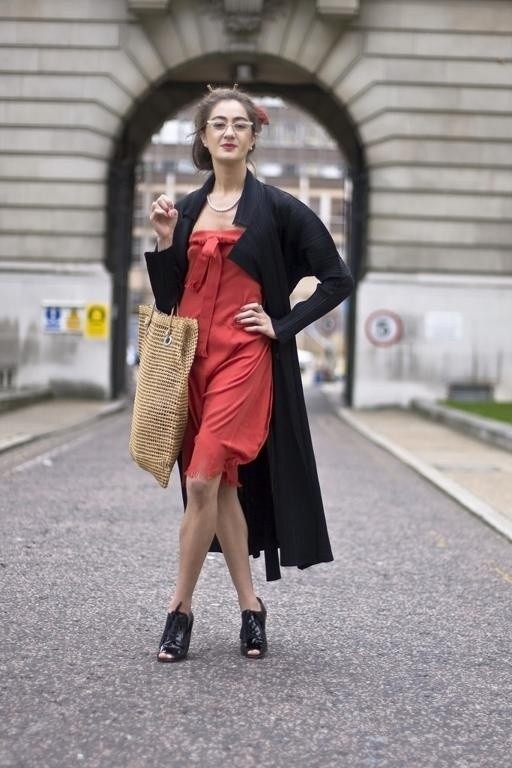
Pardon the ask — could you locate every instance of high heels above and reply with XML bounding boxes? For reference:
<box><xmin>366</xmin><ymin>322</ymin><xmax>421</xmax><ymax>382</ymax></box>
<box><xmin>239</xmin><ymin>597</ymin><xmax>268</xmax><ymax>659</ymax></box>
<box><xmin>157</xmin><ymin>601</ymin><xmax>195</xmax><ymax>663</ymax></box>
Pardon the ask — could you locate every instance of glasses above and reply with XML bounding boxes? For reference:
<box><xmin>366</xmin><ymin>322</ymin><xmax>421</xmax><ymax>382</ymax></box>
<box><xmin>206</xmin><ymin>121</ymin><xmax>254</xmax><ymax>134</ymax></box>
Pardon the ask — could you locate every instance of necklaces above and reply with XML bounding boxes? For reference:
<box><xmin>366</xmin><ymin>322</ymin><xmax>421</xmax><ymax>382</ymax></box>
<box><xmin>206</xmin><ymin>195</ymin><xmax>242</xmax><ymax>212</ymax></box>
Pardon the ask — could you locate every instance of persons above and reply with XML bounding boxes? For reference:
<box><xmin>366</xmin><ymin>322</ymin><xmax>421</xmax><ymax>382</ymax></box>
<box><xmin>150</xmin><ymin>87</ymin><xmax>355</xmax><ymax>663</ymax></box>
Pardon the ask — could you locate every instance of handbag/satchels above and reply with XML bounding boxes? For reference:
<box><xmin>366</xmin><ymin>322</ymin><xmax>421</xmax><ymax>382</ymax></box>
<box><xmin>129</xmin><ymin>305</ymin><xmax>198</xmax><ymax>490</ymax></box>
